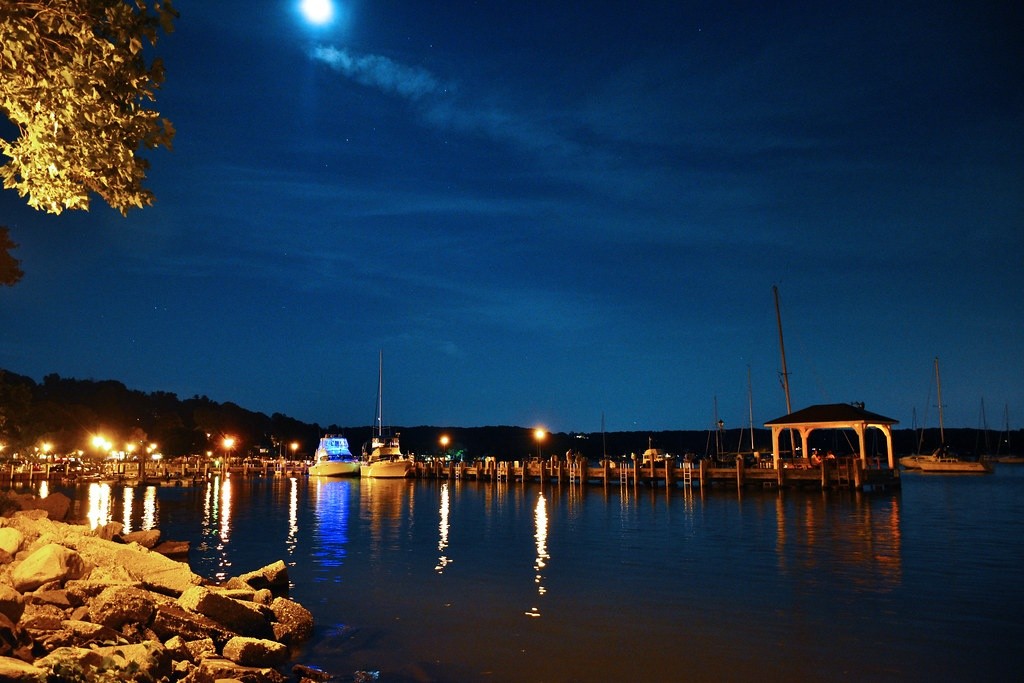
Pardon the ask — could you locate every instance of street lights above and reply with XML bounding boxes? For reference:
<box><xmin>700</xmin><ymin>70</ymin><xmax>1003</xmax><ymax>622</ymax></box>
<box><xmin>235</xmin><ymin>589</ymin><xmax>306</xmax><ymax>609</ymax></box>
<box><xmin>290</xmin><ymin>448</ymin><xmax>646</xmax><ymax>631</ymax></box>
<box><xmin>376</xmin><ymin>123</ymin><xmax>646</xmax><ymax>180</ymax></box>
<box><xmin>441</xmin><ymin>435</ymin><xmax>449</xmax><ymax>467</ymax></box>
<box><xmin>535</xmin><ymin>430</ymin><xmax>544</xmax><ymax>460</ymax></box>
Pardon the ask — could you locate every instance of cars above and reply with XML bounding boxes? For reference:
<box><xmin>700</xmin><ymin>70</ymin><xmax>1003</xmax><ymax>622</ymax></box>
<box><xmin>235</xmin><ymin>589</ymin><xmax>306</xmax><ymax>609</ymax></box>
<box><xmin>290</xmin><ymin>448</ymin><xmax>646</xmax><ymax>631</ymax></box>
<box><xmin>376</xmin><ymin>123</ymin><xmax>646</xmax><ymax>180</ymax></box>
<box><xmin>2</xmin><ymin>460</ymin><xmax>89</xmax><ymax>473</ymax></box>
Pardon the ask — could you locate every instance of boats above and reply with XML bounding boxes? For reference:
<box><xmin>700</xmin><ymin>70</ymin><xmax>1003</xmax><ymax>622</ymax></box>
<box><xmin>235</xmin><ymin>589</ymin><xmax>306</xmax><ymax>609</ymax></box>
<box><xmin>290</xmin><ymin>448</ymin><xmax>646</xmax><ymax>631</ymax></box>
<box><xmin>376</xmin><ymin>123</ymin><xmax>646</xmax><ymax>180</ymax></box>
<box><xmin>307</xmin><ymin>435</ymin><xmax>360</xmax><ymax>476</ymax></box>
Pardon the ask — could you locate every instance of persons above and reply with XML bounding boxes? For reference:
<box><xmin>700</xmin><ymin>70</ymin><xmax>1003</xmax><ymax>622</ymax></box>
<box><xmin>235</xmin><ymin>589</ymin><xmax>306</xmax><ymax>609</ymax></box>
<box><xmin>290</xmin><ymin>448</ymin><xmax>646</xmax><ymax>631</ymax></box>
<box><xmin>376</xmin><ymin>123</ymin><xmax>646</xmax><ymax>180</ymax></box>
<box><xmin>810</xmin><ymin>450</ymin><xmax>822</xmax><ymax>466</ymax></box>
<box><xmin>154</xmin><ymin>464</ymin><xmax>212</xmax><ymax>488</ymax></box>
<box><xmin>143</xmin><ymin>472</ymin><xmax>150</xmax><ymax>483</ymax></box>
<box><xmin>552</xmin><ymin>454</ymin><xmax>558</xmax><ymax>468</ymax></box>
<box><xmin>118</xmin><ymin>475</ymin><xmax>126</xmax><ymax>483</ymax></box>
<box><xmin>566</xmin><ymin>448</ymin><xmax>572</xmax><ymax>464</ymax></box>
<box><xmin>825</xmin><ymin>450</ymin><xmax>839</xmax><ymax>464</ymax></box>
<box><xmin>932</xmin><ymin>448</ymin><xmax>941</xmax><ymax>460</ymax></box>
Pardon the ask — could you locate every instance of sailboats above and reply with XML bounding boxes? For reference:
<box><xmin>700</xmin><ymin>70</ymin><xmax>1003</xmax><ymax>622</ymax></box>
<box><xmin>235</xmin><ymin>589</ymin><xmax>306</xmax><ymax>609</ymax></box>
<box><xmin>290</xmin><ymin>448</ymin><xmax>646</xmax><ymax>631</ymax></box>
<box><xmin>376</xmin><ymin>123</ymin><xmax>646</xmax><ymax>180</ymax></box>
<box><xmin>896</xmin><ymin>357</ymin><xmax>997</xmax><ymax>474</ymax></box>
<box><xmin>360</xmin><ymin>349</ymin><xmax>412</xmax><ymax>480</ymax></box>
<box><xmin>953</xmin><ymin>397</ymin><xmax>1024</xmax><ymax>463</ymax></box>
<box><xmin>599</xmin><ymin>361</ymin><xmax>838</xmax><ymax>469</ymax></box>
